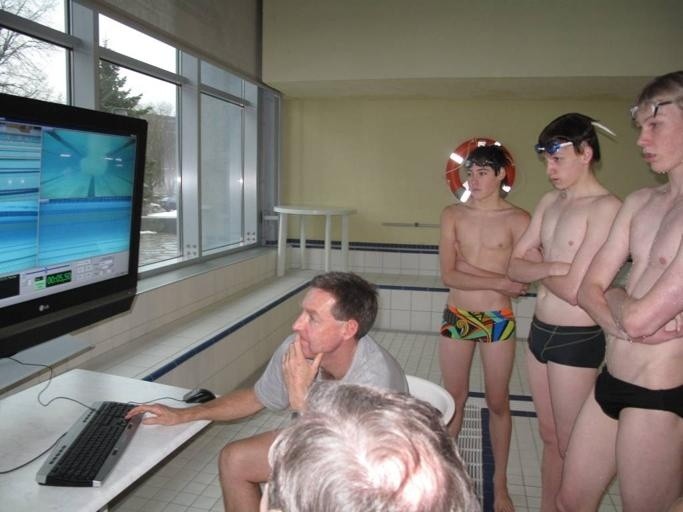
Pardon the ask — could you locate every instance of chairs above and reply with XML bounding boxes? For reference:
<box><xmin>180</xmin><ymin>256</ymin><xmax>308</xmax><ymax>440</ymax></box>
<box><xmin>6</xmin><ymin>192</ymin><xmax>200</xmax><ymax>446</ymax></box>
<box><xmin>399</xmin><ymin>371</ymin><xmax>455</xmax><ymax>430</ymax></box>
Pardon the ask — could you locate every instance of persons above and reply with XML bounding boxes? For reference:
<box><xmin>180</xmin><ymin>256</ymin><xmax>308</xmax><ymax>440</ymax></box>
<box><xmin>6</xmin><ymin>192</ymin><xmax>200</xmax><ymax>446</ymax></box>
<box><xmin>553</xmin><ymin>67</ymin><xmax>683</xmax><ymax>510</ymax></box>
<box><xmin>255</xmin><ymin>378</ymin><xmax>486</xmax><ymax>512</ymax></box>
<box><xmin>506</xmin><ymin>111</ymin><xmax>626</xmax><ymax>512</ymax></box>
<box><xmin>436</xmin><ymin>143</ymin><xmax>537</xmax><ymax>512</ymax></box>
<box><xmin>121</xmin><ymin>269</ymin><xmax>412</xmax><ymax>512</ymax></box>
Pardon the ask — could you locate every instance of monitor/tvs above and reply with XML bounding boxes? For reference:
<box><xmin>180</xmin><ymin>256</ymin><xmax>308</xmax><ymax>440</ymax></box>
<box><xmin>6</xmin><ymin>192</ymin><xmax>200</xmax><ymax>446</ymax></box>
<box><xmin>0</xmin><ymin>92</ymin><xmax>149</xmax><ymax>359</ymax></box>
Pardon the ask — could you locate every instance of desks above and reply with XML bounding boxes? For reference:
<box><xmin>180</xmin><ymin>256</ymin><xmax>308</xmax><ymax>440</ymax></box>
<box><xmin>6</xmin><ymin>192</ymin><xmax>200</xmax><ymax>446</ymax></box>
<box><xmin>0</xmin><ymin>369</ymin><xmax>222</xmax><ymax>512</ymax></box>
<box><xmin>271</xmin><ymin>198</ymin><xmax>358</xmax><ymax>280</ymax></box>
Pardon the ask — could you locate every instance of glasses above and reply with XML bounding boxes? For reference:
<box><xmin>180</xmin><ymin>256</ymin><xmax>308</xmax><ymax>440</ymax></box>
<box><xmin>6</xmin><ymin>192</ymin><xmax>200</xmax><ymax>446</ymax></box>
<box><xmin>466</xmin><ymin>157</ymin><xmax>495</xmax><ymax>167</ymax></box>
<box><xmin>629</xmin><ymin>101</ymin><xmax>673</xmax><ymax>120</ymax></box>
<box><xmin>534</xmin><ymin>143</ymin><xmax>572</xmax><ymax>154</ymax></box>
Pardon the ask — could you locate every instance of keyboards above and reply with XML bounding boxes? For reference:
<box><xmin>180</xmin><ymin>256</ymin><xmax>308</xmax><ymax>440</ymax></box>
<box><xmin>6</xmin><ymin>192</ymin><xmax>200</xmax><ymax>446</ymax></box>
<box><xmin>35</xmin><ymin>401</ymin><xmax>146</xmax><ymax>486</ymax></box>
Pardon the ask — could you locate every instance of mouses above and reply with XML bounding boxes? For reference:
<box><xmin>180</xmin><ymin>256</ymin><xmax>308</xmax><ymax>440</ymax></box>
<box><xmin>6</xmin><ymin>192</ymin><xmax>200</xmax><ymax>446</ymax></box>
<box><xmin>183</xmin><ymin>387</ymin><xmax>216</xmax><ymax>404</ymax></box>
